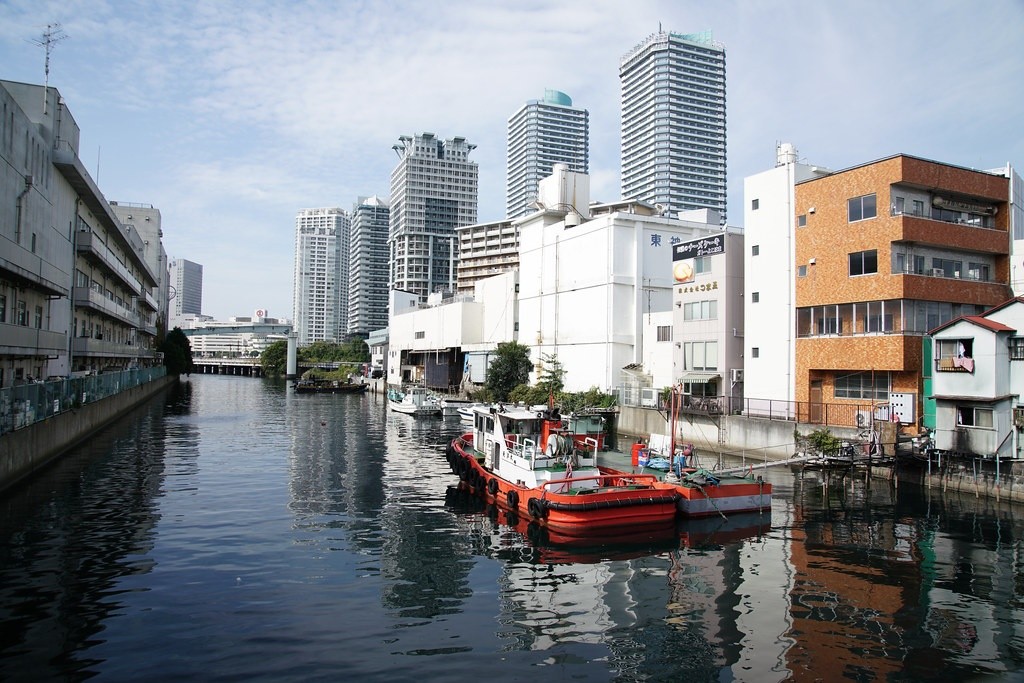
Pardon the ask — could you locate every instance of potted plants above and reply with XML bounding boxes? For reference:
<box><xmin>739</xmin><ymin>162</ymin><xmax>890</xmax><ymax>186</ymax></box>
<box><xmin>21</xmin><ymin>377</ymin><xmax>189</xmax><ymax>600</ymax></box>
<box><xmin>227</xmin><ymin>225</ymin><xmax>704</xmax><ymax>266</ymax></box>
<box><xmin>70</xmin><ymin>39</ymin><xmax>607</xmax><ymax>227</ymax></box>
<box><xmin>660</xmin><ymin>387</ymin><xmax>679</xmax><ymax>409</ymax></box>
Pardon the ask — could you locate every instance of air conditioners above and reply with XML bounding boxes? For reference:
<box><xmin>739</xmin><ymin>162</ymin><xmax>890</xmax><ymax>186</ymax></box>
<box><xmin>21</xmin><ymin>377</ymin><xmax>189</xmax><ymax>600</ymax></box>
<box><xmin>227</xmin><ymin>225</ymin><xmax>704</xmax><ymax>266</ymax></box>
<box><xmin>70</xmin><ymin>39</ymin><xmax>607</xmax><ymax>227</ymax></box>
<box><xmin>928</xmin><ymin>268</ymin><xmax>944</xmax><ymax>277</ymax></box>
<box><xmin>729</xmin><ymin>368</ymin><xmax>744</xmax><ymax>383</ymax></box>
<box><xmin>855</xmin><ymin>410</ymin><xmax>870</xmax><ymax>427</ymax></box>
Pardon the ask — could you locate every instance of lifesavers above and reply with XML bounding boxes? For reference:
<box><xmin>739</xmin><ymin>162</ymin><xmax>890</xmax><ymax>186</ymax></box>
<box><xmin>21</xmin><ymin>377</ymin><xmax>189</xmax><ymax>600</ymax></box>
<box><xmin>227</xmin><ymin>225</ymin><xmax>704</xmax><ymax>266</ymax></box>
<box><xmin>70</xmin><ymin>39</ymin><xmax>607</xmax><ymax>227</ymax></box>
<box><xmin>488</xmin><ymin>478</ymin><xmax>498</xmax><ymax>494</ymax></box>
<box><xmin>448</xmin><ymin>446</ymin><xmax>471</xmax><ymax>481</ymax></box>
<box><xmin>468</xmin><ymin>468</ymin><xmax>479</xmax><ymax>487</ymax></box>
<box><xmin>475</xmin><ymin>476</ymin><xmax>486</xmax><ymax>491</ymax></box>
<box><xmin>527</xmin><ymin>497</ymin><xmax>546</xmax><ymax>518</ymax></box>
<box><xmin>506</xmin><ymin>490</ymin><xmax>517</xmax><ymax>508</ymax></box>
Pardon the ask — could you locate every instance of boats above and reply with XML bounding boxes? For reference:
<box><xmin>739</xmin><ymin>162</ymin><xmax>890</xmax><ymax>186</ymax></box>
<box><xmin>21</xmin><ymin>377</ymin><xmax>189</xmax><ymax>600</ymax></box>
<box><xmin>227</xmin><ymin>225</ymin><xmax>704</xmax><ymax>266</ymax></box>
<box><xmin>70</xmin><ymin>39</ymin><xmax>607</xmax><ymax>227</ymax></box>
<box><xmin>389</xmin><ymin>388</ymin><xmax>441</xmax><ymax>415</ymax></box>
<box><xmin>295</xmin><ymin>381</ymin><xmax>369</xmax><ymax>393</ymax></box>
<box><xmin>446</xmin><ymin>403</ymin><xmax>682</xmax><ymax>536</ymax></box>
<box><xmin>456</xmin><ymin>403</ymin><xmax>485</xmax><ymax>420</ymax></box>
